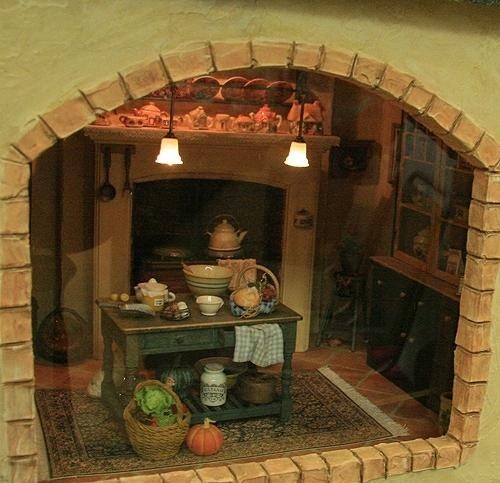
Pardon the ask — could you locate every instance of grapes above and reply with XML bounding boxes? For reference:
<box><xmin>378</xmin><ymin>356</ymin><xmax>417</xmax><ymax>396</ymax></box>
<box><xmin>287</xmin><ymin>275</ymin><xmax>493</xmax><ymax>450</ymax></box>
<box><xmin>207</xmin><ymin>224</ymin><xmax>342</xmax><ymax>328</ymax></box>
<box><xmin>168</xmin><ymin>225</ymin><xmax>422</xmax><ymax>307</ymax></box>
<box><xmin>263</xmin><ymin>284</ymin><xmax>276</xmax><ymax>302</ymax></box>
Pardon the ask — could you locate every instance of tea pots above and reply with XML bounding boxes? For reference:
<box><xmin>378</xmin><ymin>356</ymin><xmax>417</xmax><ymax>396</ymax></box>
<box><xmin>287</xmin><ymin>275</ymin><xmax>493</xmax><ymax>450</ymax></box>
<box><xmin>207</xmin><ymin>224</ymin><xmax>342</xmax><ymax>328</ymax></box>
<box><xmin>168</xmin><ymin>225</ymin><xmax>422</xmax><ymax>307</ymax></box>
<box><xmin>249</xmin><ymin>104</ymin><xmax>285</xmax><ymax>133</ymax></box>
<box><xmin>187</xmin><ymin>106</ymin><xmax>214</xmax><ymax>130</ymax></box>
<box><xmin>134</xmin><ymin>101</ymin><xmax>168</xmax><ymax>127</ymax></box>
<box><xmin>203</xmin><ymin>213</ymin><xmax>247</xmax><ymax>251</ymax></box>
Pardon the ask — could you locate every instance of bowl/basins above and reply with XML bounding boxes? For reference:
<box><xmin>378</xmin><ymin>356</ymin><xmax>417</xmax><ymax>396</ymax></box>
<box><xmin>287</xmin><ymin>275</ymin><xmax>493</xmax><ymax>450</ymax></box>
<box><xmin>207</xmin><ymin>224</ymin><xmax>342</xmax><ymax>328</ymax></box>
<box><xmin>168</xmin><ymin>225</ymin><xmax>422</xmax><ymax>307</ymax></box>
<box><xmin>183</xmin><ymin>262</ymin><xmax>235</xmax><ymax>298</ymax></box>
<box><xmin>194</xmin><ymin>356</ymin><xmax>248</xmax><ymax>389</ymax></box>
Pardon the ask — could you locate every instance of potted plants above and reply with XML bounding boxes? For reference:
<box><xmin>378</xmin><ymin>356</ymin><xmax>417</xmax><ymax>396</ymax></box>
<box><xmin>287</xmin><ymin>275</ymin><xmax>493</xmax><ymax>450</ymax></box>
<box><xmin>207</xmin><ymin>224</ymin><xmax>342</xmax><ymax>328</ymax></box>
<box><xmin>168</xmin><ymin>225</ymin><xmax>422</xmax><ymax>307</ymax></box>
<box><xmin>336</xmin><ymin>238</ymin><xmax>366</xmax><ymax>274</ymax></box>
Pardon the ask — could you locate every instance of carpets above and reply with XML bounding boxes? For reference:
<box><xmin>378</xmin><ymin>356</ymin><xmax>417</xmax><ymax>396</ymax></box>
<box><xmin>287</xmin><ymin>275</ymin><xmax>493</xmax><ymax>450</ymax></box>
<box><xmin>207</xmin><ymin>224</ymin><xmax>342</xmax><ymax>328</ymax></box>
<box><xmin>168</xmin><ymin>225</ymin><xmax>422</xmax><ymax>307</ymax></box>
<box><xmin>33</xmin><ymin>363</ymin><xmax>411</xmax><ymax>482</ymax></box>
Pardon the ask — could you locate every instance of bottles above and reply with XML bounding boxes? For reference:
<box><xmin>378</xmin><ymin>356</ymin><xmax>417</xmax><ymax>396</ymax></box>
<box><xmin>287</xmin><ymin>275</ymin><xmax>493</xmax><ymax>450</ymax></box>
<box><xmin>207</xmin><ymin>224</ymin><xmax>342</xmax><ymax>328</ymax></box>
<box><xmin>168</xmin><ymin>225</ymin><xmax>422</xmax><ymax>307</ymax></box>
<box><xmin>199</xmin><ymin>363</ymin><xmax>228</xmax><ymax>407</ymax></box>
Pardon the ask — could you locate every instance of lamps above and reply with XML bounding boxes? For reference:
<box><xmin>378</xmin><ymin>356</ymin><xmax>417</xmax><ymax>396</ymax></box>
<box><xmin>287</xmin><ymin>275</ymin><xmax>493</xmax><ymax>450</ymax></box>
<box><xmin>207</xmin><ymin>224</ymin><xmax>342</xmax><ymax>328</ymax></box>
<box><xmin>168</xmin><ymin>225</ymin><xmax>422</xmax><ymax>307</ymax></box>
<box><xmin>154</xmin><ymin>84</ymin><xmax>183</xmax><ymax>167</ymax></box>
<box><xmin>283</xmin><ymin>72</ymin><xmax>311</xmax><ymax>170</ymax></box>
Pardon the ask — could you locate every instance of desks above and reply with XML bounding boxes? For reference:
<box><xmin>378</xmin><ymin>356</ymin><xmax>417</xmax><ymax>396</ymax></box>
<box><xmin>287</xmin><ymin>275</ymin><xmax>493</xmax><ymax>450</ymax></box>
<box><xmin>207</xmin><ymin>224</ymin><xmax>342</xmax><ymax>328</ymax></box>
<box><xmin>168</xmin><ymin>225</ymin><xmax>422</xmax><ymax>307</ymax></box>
<box><xmin>314</xmin><ymin>273</ymin><xmax>369</xmax><ymax>353</ymax></box>
<box><xmin>93</xmin><ymin>294</ymin><xmax>304</xmax><ymax>426</ymax></box>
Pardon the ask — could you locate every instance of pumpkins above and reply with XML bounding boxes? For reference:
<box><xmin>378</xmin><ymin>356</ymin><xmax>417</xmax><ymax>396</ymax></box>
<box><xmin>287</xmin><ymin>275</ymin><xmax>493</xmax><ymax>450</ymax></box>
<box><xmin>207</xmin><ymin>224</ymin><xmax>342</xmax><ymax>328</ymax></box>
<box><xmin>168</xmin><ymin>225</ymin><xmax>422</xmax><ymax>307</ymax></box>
<box><xmin>185</xmin><ymin>418</ymin><xmax>223</xmax><ymax>456</ymax></box>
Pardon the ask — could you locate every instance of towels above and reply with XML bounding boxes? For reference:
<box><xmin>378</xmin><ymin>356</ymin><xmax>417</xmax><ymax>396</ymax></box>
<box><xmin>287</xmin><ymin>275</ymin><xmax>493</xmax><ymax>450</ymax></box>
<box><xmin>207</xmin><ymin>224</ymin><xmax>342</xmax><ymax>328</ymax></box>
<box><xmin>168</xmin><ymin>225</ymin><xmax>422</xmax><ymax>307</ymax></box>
<box><xmin>231</xmin><ymin>324</ymin><xmax>286</xmax><ymax>368</ymax></box>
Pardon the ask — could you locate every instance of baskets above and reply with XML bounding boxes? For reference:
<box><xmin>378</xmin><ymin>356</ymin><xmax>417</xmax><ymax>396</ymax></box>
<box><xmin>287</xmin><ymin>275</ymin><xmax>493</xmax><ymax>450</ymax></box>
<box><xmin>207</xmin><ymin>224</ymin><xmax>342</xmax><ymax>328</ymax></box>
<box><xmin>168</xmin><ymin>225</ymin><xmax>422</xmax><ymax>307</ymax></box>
<box><xmin>124</xmin><ymin>381</ymin><xmax>192</xmax><ymax>461</ymax></box>
<box><xmin>36</xmin><ymin>308</ymin><xmax>93</xmax><ymax>363</ymax></box>
<box><xmin>230</xmin><ymin>266</ymin><xmax>279</xmax><ymax>316</ymax></box>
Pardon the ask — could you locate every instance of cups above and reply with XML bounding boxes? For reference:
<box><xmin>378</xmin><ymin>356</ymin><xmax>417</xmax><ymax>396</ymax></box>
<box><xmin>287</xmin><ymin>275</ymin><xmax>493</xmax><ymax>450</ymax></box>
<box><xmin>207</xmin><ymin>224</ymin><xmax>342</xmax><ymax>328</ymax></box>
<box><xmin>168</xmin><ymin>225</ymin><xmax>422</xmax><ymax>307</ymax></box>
<box><xmin>162</xmin><ymin>116</ymin><xmax>183</xmax><ymax>128</ymax></box>
<box><xmin>119</xmin><ymin>114</ymin><xmax>145</xmax><ymax>127</ymax></box>
<box><xmin>133</xmin><ymin>280</ymin><xmax>176</xmax><ymax>306</ymax></box>
<box><xmin>216</xmin><ymin>113</ymin><xmax>236</xmax><ymax>131</ymax></box>
<box><xmin>236</xmin><ymin>115</ymin><xmax>256</xmax><ymax>132</ymax></box>
<box><xmin>196</xmin><ymin>295</ymin><xmax>224</xmax><ymax>316</ymax></box>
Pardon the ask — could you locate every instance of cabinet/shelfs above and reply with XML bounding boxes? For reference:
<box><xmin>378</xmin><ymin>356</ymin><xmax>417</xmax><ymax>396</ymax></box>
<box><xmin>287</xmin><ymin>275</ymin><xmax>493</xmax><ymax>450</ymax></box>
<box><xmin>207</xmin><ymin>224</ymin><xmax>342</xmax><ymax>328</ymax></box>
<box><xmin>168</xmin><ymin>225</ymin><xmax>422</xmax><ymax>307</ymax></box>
<box><xmin>365</xmin><ymin>111</ymin><xmax>476</xmax><ymax>413</ymax></box>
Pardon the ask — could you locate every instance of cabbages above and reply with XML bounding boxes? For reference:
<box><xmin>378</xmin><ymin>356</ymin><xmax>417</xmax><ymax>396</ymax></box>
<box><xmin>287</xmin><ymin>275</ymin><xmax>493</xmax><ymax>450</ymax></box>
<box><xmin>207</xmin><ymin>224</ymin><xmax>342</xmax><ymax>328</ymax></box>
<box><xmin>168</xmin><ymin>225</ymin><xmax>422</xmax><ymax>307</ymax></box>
<box><xmin>134</xmin><ymin>383</ymin><xmax>176</xmax><ymax>415</ymax></box>
